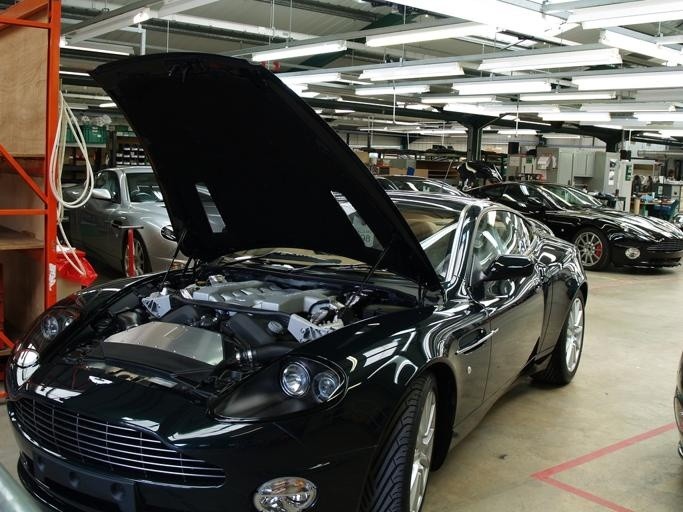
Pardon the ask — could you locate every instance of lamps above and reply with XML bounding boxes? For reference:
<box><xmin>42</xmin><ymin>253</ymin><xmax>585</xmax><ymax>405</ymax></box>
<box><xmin>62</xmin><ymin>0</ymin><xmax>682</xmax><ymax>141</ymax></box>
